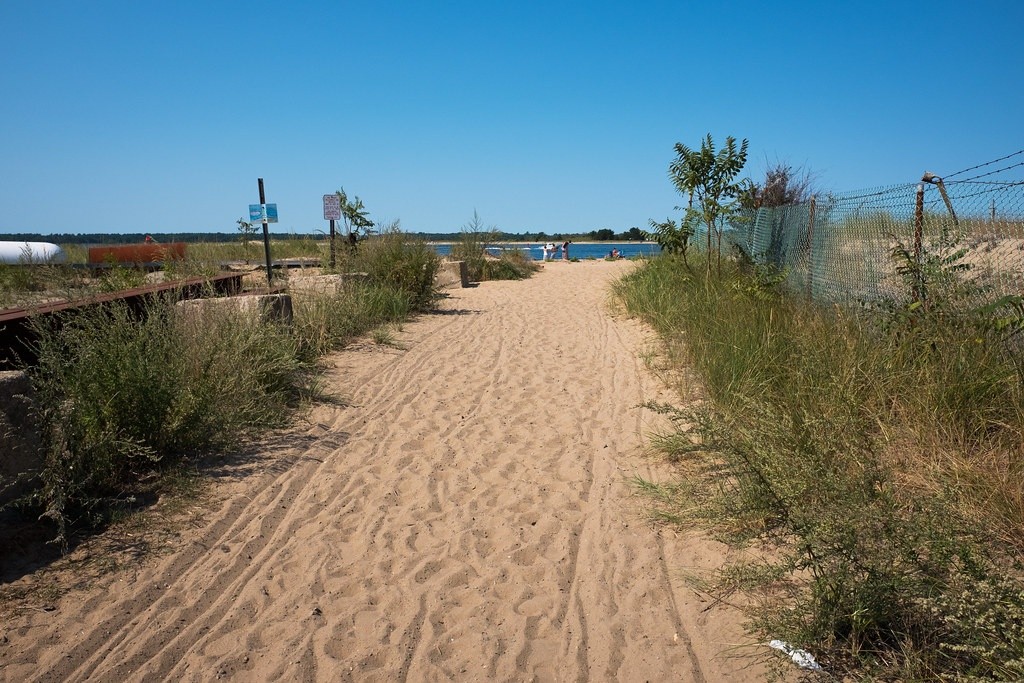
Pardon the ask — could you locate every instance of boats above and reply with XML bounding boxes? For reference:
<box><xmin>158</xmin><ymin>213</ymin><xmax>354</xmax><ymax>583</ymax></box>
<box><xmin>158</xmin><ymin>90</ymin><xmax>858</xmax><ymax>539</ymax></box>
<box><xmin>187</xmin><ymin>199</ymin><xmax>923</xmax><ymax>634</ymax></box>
<box><xmin>540</xmin><ymin>243</ymin><xmax>562</xmax><ymax>252</ymax></box>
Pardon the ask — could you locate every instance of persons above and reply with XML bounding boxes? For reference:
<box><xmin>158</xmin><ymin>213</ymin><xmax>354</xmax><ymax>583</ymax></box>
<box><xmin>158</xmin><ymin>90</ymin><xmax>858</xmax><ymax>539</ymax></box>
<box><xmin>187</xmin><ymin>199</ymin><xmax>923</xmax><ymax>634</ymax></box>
<box><xmin>612</xmin><ymin>247</ymin><xmax>617</xmax><ymax>256</ymax></box>
<box><xmin>615</xmin><ymin>250</ymin><xmax>622</xmax><ymax>258</ymax></box>
<box><xmin>561</xmin><ymin>240</ymin><xmax>571</xmax><ymax>260</ymax></box>
<box><xmin>543</xmin><ymin>243</ymin><xmax>557</xmax><ymax>259</ymax></box>
